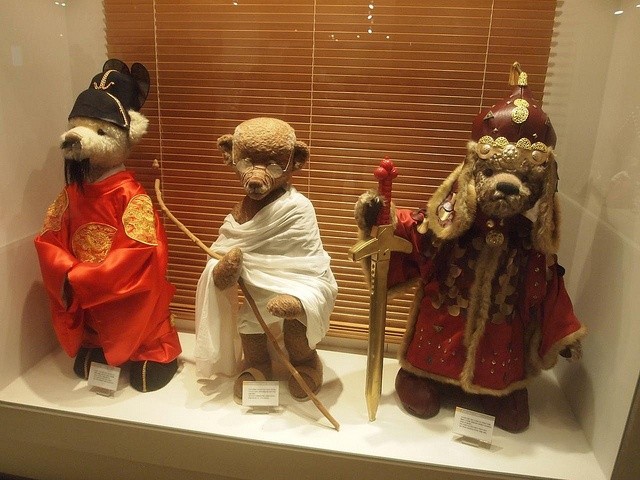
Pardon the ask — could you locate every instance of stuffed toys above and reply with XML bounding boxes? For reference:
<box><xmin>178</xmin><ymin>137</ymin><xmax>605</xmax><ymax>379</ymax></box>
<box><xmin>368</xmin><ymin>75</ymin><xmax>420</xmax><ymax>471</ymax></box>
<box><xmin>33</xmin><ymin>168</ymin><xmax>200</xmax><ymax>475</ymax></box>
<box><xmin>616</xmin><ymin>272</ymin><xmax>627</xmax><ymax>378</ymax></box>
<box><xmin>353</xmin><ymin>60</ymin><xmax>585</xmax><ymax>432</ymax></box>
<box><xmin>33</xmin><ymin>60</ymin><xmax>183</xmax><ymax>395</ymax></box>
<box><xmin>196</xmin><ymin>116</ymin><xmax>339</xmax><ymax>405</ymax></box>
